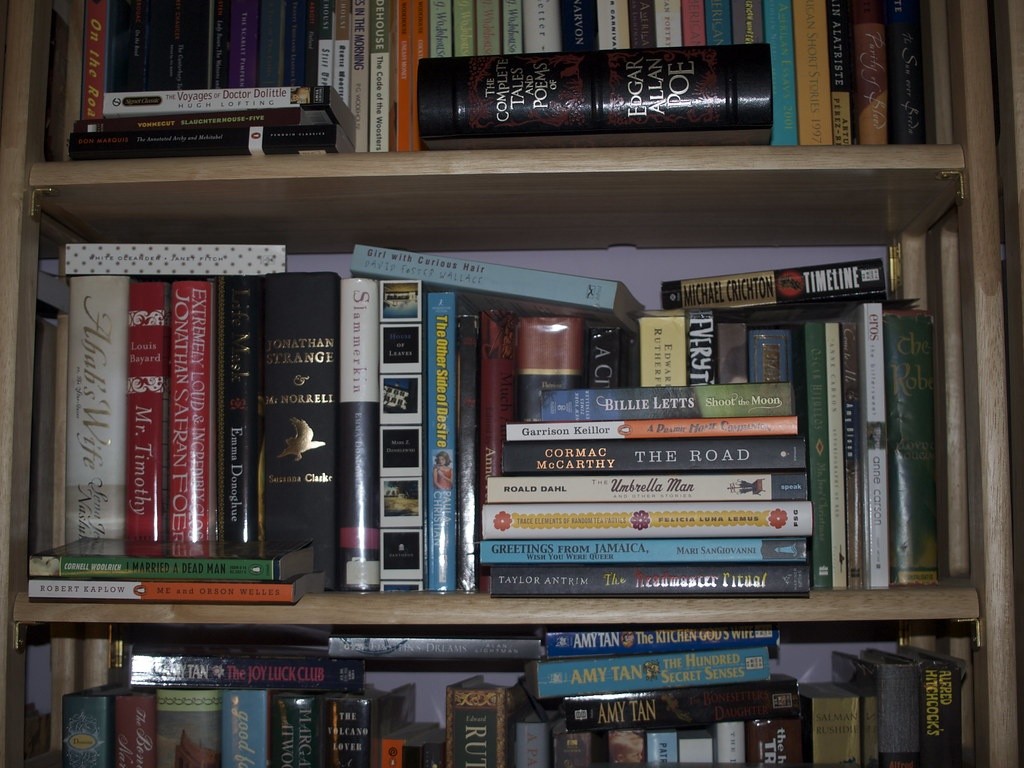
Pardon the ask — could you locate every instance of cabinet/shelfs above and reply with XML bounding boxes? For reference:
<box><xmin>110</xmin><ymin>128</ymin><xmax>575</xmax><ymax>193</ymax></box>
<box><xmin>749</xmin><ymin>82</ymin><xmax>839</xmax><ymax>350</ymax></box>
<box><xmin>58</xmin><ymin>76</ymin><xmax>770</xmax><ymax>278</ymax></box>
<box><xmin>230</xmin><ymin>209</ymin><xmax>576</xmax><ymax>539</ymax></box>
<box><xmin>2</xmin><ymin>2</ymin><xmax>1024</xmax><ymax>765</ymax></box>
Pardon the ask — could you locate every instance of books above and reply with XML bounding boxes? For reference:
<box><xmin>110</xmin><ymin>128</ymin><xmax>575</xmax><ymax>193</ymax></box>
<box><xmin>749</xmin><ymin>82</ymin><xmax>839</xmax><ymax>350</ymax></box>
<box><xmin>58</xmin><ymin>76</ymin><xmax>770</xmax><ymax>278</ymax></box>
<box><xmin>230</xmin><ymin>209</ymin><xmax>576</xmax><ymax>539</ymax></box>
<box><xmin>43</xmin><ymin>623</ymin><xmax>983</xmax><ymax>768</ymax></box>
<box><xmin>25</xmin><ymin>230</ymin><xmax>971</xmax><ymax>609</ymax></box>
<box><xmin>64</xmin><ymin>1</ymin><xmax>927</xmax><ymax>159</ymax></box>
